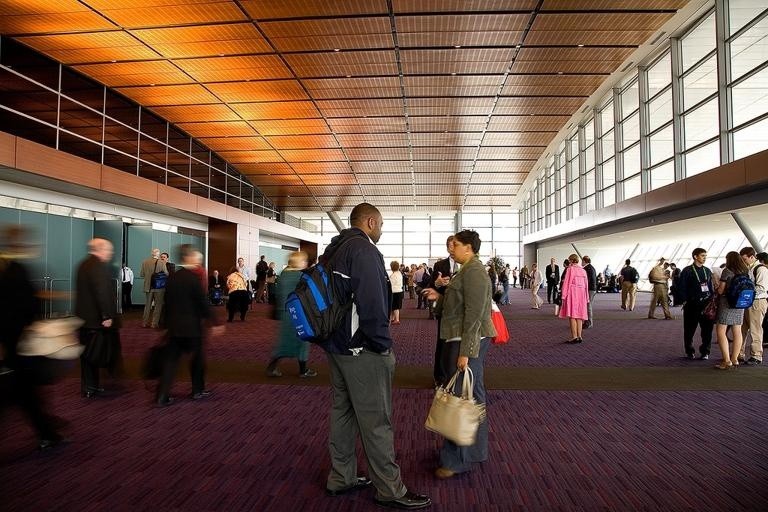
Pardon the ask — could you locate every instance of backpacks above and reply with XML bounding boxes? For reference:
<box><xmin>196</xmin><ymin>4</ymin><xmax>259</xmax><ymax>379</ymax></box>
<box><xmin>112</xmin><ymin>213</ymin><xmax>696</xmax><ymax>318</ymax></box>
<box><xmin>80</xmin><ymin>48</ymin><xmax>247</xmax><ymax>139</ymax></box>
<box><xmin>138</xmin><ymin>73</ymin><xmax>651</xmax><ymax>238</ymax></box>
<box><xmin>726</xmin><ymin>272</ymin><xmax>756</xmax><ymax>309</ymax></box>
<box><xmin>500</xmin><ymin>270</ymin><xmax>508</xmax><ymax>282</ymax></box>
<box><xmin>286</xmin><ymin>236</ymin><xmax>370</xmax><ymax>344</ymax></box>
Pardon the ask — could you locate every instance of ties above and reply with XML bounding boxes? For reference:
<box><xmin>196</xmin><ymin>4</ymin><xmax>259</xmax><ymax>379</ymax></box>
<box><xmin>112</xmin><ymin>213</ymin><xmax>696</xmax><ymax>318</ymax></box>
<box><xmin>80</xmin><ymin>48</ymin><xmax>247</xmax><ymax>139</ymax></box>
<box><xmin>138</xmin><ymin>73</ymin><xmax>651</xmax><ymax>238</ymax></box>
<box><xmin>123</xmin><ymin>269</ymin><xmax>125</xmax><ymax>281</ymax></box>
<box><xmin>453</xmin><ymin>262</ymin><xmax>458</xmax><ymax>272</ymax></box>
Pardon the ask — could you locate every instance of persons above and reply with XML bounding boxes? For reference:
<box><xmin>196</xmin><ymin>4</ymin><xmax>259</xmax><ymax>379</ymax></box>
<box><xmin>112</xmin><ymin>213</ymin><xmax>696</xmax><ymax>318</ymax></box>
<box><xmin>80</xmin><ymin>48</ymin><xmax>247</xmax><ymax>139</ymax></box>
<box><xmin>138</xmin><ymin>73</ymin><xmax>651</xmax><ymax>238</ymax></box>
<box><xmin>140</xmin><ymin>246</ymin><xmax>322</xmax><ymax>402</ymax></box>
<box><xmin>430</xmin><ymin>235</ymin><xmax>462</xmax><ymax>320</ymax></box>
<box><xmin>421</xmin><ymin>229</ymin><xmax>499</xmax><ymax>480</ymax></box>
<box><xmin>72</xmin><ymin>238</ymin><xmax>122</xmax><ymax>400</ymax></box>
<box><xmin>620</xmin><ymin>259</ymin><xmax>639</xmax><ymax>311</ymax></box>
<box><xmin>649</xmin><ymin>247</ymin><xmax>768</xmax><ymax>370</ymax></box>
<box><xmin>389</xmin><ymin>261</ymin><xmax>433</xmax><ymax>325</ymax></box>
<box><xmin>119</xmin><ymin>261</ymin><xmax>134</xmax><ymax>311</ymax></box>
<box><xmin>499</xmin><ymin>254</ymin><xmax>596</xmax><ymax>344</ymax></box>
<box><xmin>76</xmin><ymin>238</ymin><xmax>120</xmax><ymax>398</ymax></box>
<box><xmin>318</xmin><ymin>202</ymin><xmax>432</xmax><ymax>509</ymax></box>
<box><xmin>0</xmin><ymin>222</ymin><xmax>71</xmax><ymax>463</ymax></box>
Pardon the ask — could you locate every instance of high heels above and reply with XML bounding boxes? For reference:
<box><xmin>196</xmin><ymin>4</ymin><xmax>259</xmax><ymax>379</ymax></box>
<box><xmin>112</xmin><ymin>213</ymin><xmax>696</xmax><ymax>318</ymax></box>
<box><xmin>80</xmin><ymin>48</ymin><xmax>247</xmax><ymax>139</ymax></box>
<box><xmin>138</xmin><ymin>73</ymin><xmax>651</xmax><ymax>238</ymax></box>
<box><xmin>714</xmin><ymin>360</ymin><xmax>740</xmax><ymax>371</ymax></box>
<box><xmin>565</xmin><ymin>337</ymin><xmax>583</xmax><ymax>344</ymax></box>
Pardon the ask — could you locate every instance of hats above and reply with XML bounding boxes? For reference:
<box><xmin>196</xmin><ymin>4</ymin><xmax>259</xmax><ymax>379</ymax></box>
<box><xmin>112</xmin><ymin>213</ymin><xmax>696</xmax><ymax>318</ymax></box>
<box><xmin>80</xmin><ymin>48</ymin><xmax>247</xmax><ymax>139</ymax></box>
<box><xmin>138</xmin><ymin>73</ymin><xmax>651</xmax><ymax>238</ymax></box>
<box><xmin>283</xmin><ymin>251</ymin><xmax>308</xmax><ymax>271</ymax></box>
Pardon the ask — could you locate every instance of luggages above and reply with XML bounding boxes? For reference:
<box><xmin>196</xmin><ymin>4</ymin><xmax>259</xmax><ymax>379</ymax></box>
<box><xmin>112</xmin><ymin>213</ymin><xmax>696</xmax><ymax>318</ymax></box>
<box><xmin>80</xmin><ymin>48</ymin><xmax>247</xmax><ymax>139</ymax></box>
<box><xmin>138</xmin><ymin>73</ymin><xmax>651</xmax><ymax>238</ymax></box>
<box><xmin>494</xmin><ymin>291</ymin><xmax>503</xmax><ymax>304</ymax></box>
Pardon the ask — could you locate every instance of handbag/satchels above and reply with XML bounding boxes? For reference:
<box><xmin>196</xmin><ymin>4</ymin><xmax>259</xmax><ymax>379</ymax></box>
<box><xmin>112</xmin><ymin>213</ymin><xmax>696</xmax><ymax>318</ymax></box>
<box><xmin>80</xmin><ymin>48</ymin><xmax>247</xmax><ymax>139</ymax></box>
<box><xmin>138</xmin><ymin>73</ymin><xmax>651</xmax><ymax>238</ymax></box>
<box><xmin>248</xmin><ymin>291</ymin><xmax>252</xmax><ymax>305</ymax></box>
<box><xmin>151</xmin><ymin>272</ymin><xmax>167</xmax><ymax>289</ymax></box>
<box><xmin>702</xmin><ymin>287</ymin><xmax>718</xmax><ymax>320</ymax></box>
<box><xmin>422</xmin><ymin>272</ymin><xmax>431</xmax><ymax>283</ymax></box>
<box><xmin>265</xmin><ymin>276</ymin><xmax>276</xmax><ymax>284</ymax></box>
<box><xmin>425</xmin><ymin>388</ymin><xmax>485</xmax><ymax>446</ymax></box>
<box><xmin>488</xmin><ymin>299</ymin><xmax>510</xmax><ymax>345</ymax></box>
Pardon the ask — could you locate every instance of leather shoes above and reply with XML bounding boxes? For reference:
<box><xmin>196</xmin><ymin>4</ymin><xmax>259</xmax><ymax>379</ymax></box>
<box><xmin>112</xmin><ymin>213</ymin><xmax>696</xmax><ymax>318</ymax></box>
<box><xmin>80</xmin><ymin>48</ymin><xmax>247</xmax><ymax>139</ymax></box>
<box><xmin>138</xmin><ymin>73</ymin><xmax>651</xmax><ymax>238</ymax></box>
<box><xmin>392</xmin><ymin>321</ymin><xmax>400</xmax><ymax>324</ymax></box>
<box><xmin>688</xmin><ymin>353</ymin><xmax>696</xmax><ymax>360</ymax></box>
<box><xmin>81</xmin><ymin>387</ymin><xmax>105</xmax><ymax>398</ymax></box>
<box><xmin>190</xmin><ymin>390</ymin><xmax>216</xmax><ymax>400</ymax></box>
<box><xmin>375</xmin><ymin>490</ymin><xmax>432</xmax><ymax>511</ymax></box>
<box><xmin>154</xmin><ymin>395</ymin><xmax>174</xmax><ymax>408</ymax></box>
<box><xmin>326</xmin><ymin>474</ymin><xmax>372</xmax><ymax>496</ymax></box>
<box><xmin>436</xmin><ymin>469</ymin><xmax>458</xmax><ymax>479</ymax></box>
<box><xmin>701</xmin><ymin>354</ymin><xmax>709</xmax><ymax>359</ymax></box>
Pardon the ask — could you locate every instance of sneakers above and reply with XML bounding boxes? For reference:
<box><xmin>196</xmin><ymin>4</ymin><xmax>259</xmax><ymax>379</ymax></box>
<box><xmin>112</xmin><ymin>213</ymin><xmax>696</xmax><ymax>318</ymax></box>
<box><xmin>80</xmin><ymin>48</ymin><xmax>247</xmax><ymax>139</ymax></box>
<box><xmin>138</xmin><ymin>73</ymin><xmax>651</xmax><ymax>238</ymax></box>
<box><xmin>746</xmin><ymin>358</ymin><xmax>761</xmax><ymax>366</ymax></box>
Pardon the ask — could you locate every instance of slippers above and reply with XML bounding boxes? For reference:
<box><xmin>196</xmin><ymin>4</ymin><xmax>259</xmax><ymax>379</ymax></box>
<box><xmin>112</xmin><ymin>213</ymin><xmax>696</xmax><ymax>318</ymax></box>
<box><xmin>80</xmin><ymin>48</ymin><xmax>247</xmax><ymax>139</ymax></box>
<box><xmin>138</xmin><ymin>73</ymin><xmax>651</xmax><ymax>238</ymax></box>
<box><xmin>266</xmin><ymin>367</ymin><xmax>282</xmax><ymax>376</ymax></box>
<box><xmin>300</xmin><ymin>368</ymin><xmax>318</xmax><ymax>378</ymax></box>
